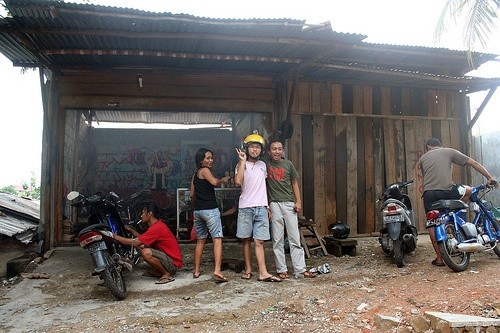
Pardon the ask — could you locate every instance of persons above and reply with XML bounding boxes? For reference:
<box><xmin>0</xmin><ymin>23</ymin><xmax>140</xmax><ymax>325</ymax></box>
<box><xmin>220</xmin><ymin>191</ymin><xmax>242</xmax><ymax>217</ymax></box>
<box><xmin>266</xmin><ymin>140</ymin><xmax>318</xmax><ymax>278</ymax></box>
<box><xmin>189</xmin><ymin>148</ymin><xmax>230</xmax><ymax>281</ymax></box>
<box><xmin>111</xmin><ymin>202</ymin><xmax>182</xmax><ymax>284</ymax></box>
<box><xmin>415</xmin><ymin>137</ymin><xmax>500</xmax><ymax>266</ymax></box>
<box><xmin>234</xmin><ymin>134</ymin><xmax>283</xmax><ymax>282</ymax></box>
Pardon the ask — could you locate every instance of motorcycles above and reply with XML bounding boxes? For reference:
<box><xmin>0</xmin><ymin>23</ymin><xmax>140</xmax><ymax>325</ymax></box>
<box><xmin>425</xmin><ymin>180</ymin><xmax>500</xmax><ymax>273</ymax></box>
<box><xmin>66</xmin><ymin>191</ymin><xmax>141</xmax><ymax>299</ymax></box>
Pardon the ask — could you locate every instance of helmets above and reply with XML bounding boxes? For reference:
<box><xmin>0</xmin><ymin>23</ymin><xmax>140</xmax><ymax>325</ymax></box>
<box><xmin>330</xmin><ymin>220</ymin><xmax>350</xmax><ymax>239</ymax></box>
<box><xmin>243</xmin><ymin>134</ymin><xmax>266</xmax><ymax>157</ymax></box>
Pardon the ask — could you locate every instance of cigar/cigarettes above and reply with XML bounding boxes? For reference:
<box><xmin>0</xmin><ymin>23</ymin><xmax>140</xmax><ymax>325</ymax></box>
<box><xmin>136</xmin><ymin>220</ymin><xmax>142</xmax><ymax>225</ymax></box>
<box><xmin>254</xmin><ymin>153</ymin><xmax>256</xmax><ymax>156</ymax></box>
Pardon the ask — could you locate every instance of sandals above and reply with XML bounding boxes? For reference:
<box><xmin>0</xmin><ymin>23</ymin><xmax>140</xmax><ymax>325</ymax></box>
<box><xmin>301</xmin><ymin>271</ymin><xmax>316</xmax><ymax>278</ymax></box>
<box><xmin>278</xmin><ymin>273</ymin><xmax>290</xmax><ymax>279</ymax></box>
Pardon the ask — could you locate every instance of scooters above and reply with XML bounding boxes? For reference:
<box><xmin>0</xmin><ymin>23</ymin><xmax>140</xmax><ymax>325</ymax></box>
<box><xmin>375</xmin><ymin>179</ymin><xmax>418</xmax><ymax>268</ymax></box>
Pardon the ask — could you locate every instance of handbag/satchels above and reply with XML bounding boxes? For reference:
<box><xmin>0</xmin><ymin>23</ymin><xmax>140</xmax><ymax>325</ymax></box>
<box><xmin>189</xmin><ymin>215</ymin><xmax>197</xmax><ymax>242</ymax></box>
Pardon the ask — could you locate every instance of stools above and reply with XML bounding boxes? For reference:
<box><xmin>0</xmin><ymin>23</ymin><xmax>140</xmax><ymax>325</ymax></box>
<box><xmin>324</xmin><ymin>235</ymin><xmax>358</xmax><ymax>258</ymax></box>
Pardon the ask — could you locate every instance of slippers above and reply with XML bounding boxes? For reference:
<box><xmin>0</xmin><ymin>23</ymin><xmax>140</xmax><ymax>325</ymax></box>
<box><xmin>193</xmin><ymin>270</ymin><xmax>202</xmax><ymax>278</ymax></box>
<box><xmin>155</xmin><ymin>277</ymin><xmax>175</xmax><ymax>284</ymax></box>
<box><xmin>215</xmin><ymin>277</ymin><xmax>227</xmax><ymax>281</ymax></box>
<box><xmin>257</xmin><ymin>275</ymin><xmax>282</xmax><ymax>282</ymax></box>
<box><xmin>142</xmin><ymin>272</ymin><xmax>162</xmax><ymax>277</ymax></box>
<box><xmin>242</xmin><ymin>271</ymin><xmax>253</xmax><ymax>279</ymax></box>
<box><xmin>432</xmin><ymin>258</ymin><xmax>445</xmax><ymax>266</ymax></box>
